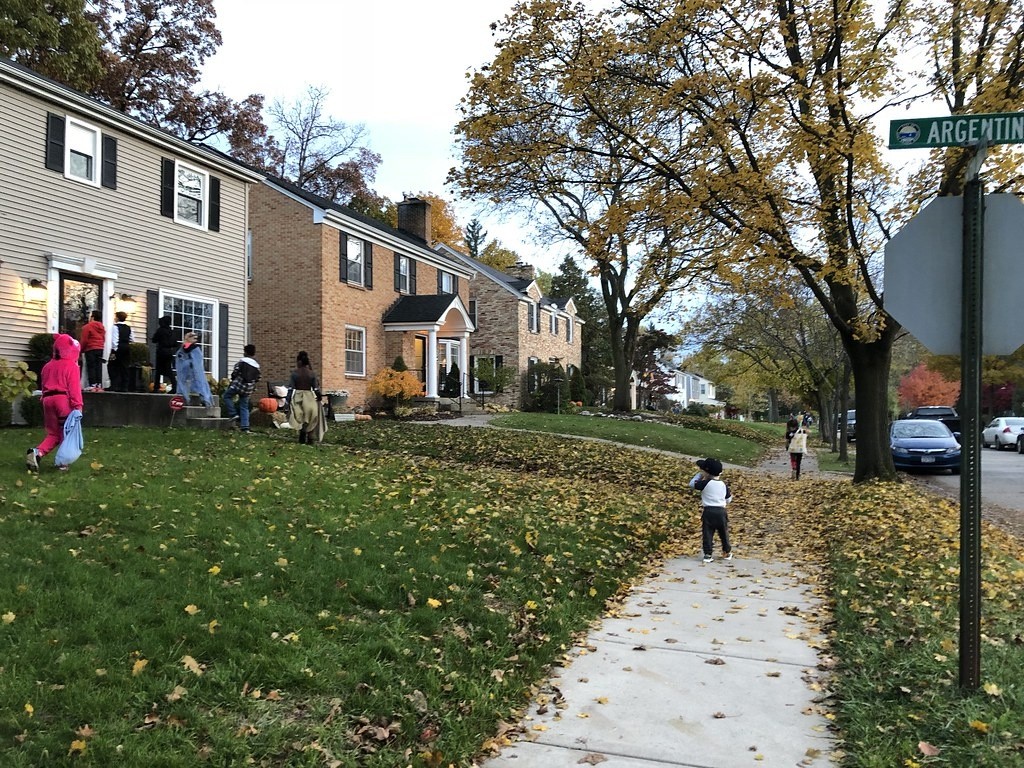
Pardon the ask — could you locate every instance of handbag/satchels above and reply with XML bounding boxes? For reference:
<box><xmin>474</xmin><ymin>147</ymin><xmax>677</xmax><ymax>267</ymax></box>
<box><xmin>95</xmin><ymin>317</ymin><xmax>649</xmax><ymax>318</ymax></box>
<box><xmin>788</xmin><ymin>428</ymin><xmax>808</xmax><ymax>455</ymax></box>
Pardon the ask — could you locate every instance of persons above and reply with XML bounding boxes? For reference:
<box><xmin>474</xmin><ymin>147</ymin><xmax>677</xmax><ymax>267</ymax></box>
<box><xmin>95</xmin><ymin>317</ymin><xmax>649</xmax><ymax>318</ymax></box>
<box><xmin>286</xmin><ymin>351</ymin><xmax>322</xmax><ymax>445</ymax></box>
<box><xmin>104</xmin><ymin>312</ymin><xmax>133</xmax><ymax>391</ymax></box>
<box><xmin>150</xmin><ymin>316</ymin><xmax>177</xmax><ymax>393</ymax></box>
<box><xmin>80</xmin><ymin>311</ymin><xmax>106</xmax><ymax>392</ymax></box>
<box><xmin>175</xmin><ymin>332</ymin><xmax>212</xmax><ymax>406</ymax></box>
<box><xmin>688</xmin><ymin>457</ymin><xmax>734</xmax><ymax>562</ymax></box>
<box><xmin>786</xmin><ymin>410</ymin><xmax>813</xmax><ymax>481</ymax></box>
<box><xmin>25</xmin><ymin>332</ymin><xmax>81</xmax><ymax>473</ymax></box>
<box><xmin>223</xmin><ymin>345</ymin><xmax>261</xmax><ymax>431</ymax></box>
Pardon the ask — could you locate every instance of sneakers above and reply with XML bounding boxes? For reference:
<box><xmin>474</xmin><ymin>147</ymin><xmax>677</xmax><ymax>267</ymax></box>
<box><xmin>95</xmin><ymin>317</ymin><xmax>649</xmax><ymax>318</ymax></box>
<box><xmin>723</xmin><ymin>551</ymin><xmax>732</xmax><ymax>559</ymax></box>
<box><xmin>703</xmin><ymin>555</ymin><xmax>714</xmax><ymax>562</ymax></box>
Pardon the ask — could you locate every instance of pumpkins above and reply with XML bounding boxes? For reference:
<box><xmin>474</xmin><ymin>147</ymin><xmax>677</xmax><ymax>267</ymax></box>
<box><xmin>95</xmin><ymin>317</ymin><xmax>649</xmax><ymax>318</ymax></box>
<box><xmin>570</xmin><ymin>401</ymin><xmax>582</xmax><ymax>406</ymax></box>
<box><xmin>259</xmin><ymin>398</ymin><xmax>277</xmax><ymax>412</ymax></box>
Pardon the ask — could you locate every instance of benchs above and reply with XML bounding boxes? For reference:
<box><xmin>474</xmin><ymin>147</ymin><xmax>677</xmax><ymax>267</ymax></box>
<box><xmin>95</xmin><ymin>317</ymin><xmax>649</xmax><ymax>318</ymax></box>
<box><xmin>266</xmin><ymin>382</ymin><xmax>336</xmax><ymax>417</ymax></box>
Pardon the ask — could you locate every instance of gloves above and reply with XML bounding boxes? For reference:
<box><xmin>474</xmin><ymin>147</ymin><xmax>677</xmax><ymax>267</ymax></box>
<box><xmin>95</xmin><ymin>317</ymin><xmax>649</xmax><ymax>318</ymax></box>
<box><xmin>282</xmin><ymin>389</ymin><xmax>293</xmax><ymax>413</ymax></box>
<box><xmin>315</xmin><ymin>390</ymin><xmax>322</xmax><ymax>401</ymax></box>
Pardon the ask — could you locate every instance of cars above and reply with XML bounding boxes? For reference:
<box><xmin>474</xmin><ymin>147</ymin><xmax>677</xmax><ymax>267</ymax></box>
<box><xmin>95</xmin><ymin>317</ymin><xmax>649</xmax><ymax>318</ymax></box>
<box><xmin>887</xmin><ymin>419</ymin><xmax>962</xmax><ymax>475</ymax></box>
<box><xmin>982</xmin><ymin>417</ymin><xmax>1024</xmax><ymax>454</ymax></box>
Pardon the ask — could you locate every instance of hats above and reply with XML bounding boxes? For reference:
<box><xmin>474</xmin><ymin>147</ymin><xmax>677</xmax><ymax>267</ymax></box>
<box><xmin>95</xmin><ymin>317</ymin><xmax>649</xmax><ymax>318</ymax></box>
<box><xmin>696</xmin><ymin>457</ymin><xmax>722</xmax><ymax>476</ymax></box>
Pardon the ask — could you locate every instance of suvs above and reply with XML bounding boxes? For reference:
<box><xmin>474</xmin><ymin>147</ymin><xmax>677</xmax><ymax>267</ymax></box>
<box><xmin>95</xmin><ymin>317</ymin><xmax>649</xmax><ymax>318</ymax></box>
<box><xmin>899</xmin><ymin>406</ymin><xmax>962</xmax><ymax>433</ymax></box>
<box><xmin>846</xmin><ymin>410</ymin><xmax>856</xmax><ymax>442</ymax></box>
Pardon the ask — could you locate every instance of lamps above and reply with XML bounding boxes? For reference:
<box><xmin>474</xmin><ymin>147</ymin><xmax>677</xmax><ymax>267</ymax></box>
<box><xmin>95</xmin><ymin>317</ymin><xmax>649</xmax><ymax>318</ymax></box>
<box><xmin>31</xmin><ymin>278</ymin><xmax>47</xmax><ymax>290</ymax></box>
<box><xmin>113</xmin><ymin>291</ymin><xmax>137</xmax><ymax>303</ymax></box>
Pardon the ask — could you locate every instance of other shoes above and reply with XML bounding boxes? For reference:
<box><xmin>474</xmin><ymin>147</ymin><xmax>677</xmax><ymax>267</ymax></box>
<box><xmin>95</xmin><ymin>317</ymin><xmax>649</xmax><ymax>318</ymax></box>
<box><xmin>241</xmin><ymin>429</ymin><xmax>250</xmax><ymax>433</ymax></box>
<box><xmin>227</xmin><ymin>414</ymin><xmax>240</xmax><ymax>423</ymax></box>
<box><xmin>60</xmin><ymin>465</ymin><xmax>69</xmax><ymax>471</ymax></box>
<box><xmin>27</xmin><ymin>448</ymin><xmax>41</xmax><ymax>473</ymax></box>
<box><xmin>90</xmin><ymin>387</ymin><xmax>104</xmax><ymax>392</ymax></box>
